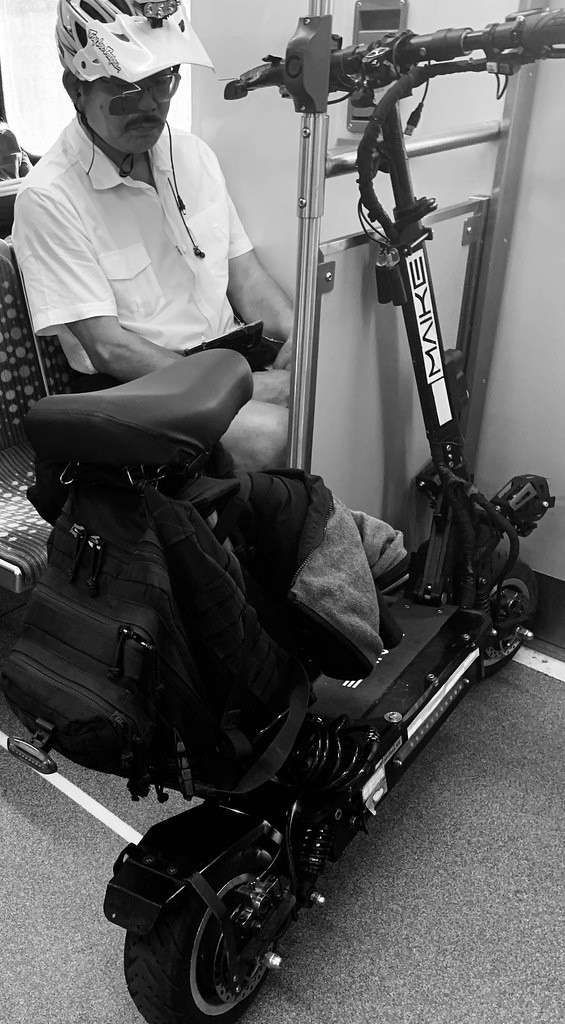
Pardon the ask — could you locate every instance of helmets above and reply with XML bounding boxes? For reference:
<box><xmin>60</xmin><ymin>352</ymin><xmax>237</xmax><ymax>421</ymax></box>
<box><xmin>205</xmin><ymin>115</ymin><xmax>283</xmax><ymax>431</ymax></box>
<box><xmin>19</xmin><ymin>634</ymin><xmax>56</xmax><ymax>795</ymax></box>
<box><xmin>56</xmin><ymin>0</ymin><xmax>216</xmax><ymax>83</ymax></box>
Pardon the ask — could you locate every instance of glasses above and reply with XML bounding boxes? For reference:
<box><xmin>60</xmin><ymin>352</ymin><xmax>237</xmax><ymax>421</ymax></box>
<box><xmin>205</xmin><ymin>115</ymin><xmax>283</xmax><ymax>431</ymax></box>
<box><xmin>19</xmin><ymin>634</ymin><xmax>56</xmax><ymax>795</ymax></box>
<box><xmin>92</xmin><ymin>71</ymin><xmax>182</xmax><ymax>103</ymax></box>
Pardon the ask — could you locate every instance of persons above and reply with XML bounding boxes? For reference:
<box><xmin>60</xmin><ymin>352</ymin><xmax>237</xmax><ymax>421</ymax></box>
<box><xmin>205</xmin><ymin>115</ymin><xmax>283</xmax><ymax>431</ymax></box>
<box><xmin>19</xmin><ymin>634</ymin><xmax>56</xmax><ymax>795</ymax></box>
<box><xmin>10</xmin><ymin>0</ymin><xmax>295</xmax><ymax>473</ymax></box>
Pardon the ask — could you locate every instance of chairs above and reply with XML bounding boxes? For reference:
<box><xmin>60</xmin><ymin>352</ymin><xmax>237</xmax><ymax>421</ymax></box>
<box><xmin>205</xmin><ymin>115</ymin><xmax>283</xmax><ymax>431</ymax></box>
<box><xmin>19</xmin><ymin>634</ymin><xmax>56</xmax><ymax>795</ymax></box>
<box><xmin>0</xmin><ymin>237</ymin><xmax>97</xmax><ymax>596</ymax></box>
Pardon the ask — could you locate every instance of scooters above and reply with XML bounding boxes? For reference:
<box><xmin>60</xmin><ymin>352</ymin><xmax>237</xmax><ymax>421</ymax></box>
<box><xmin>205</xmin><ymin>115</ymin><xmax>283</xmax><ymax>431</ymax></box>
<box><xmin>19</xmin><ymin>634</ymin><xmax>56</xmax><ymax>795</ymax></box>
<box><xmin>27</xmin><ymin>2</ymin><xmax>565</xmax><ymax>1023</ymax></box>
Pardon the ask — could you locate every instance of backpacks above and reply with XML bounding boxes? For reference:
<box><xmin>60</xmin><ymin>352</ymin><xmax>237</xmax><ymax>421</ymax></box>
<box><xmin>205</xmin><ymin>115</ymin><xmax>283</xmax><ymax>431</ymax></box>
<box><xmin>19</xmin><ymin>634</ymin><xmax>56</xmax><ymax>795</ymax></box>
<box><xmin>0</xmin><ymin>477</ymin><xmax>317</xmax><ymax>794</ymax></box>
